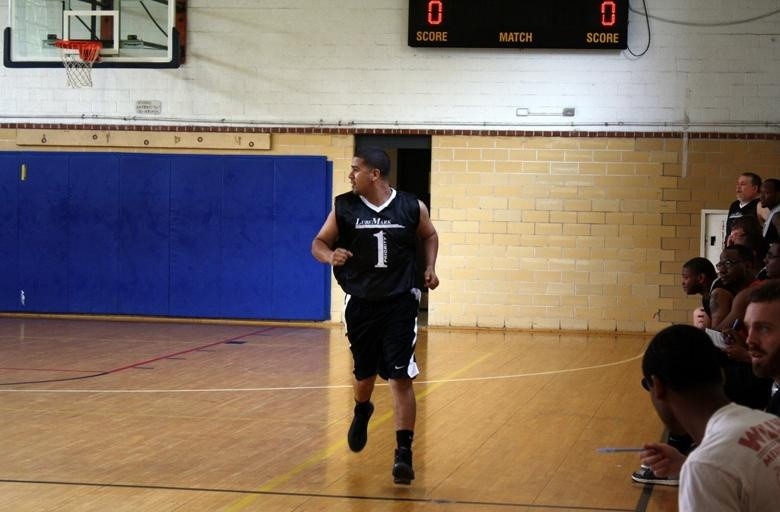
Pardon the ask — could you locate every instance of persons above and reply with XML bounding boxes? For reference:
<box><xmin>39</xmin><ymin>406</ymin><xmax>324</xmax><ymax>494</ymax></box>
<box><xmin>312</xmin><ymin>146</ymin><xmax>438</xmax><ymax>485</ymax></box>
<box><xmin>631</xmin><ymin>172</ymin><xmax>780</xmax><ymax>512</ymax></box>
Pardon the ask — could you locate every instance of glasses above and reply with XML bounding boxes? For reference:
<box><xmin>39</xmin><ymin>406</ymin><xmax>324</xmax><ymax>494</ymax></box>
<box><xmin>641</xmin><ymin>378</ymin><xmax>649</xmax><ymax>392</ymax></box>
<box><xmin>715</xmin><ymin>260</ymin><xmax>745</xmax><ymax>270</ymax></box>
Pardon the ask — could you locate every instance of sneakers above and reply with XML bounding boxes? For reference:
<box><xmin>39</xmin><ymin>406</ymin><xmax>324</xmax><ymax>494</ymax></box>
<box><xmin>348</xmin><ymin>402</ymin><xmax>374</xmax><ymax>452</ymax></box>
<box><xmin>631</xmin><ymin>470</ymin><xmax>680</xmax><ymax>486</ymax></box>
<box><xmin>393</xmin><ymin>448</ymin><xmax>415</xmax><ymax>485</ymax></box>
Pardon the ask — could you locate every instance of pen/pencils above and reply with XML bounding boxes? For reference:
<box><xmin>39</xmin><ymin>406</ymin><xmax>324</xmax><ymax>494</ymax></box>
<box><xmin>597</xmin><ymin>448</ymin><xmax>651</xmax><ymax>452</ymax></box>
<box><xmin>732</xmin><ymin>319</ymin><xmax>738</xmax><ymax>329</ymax></box>
